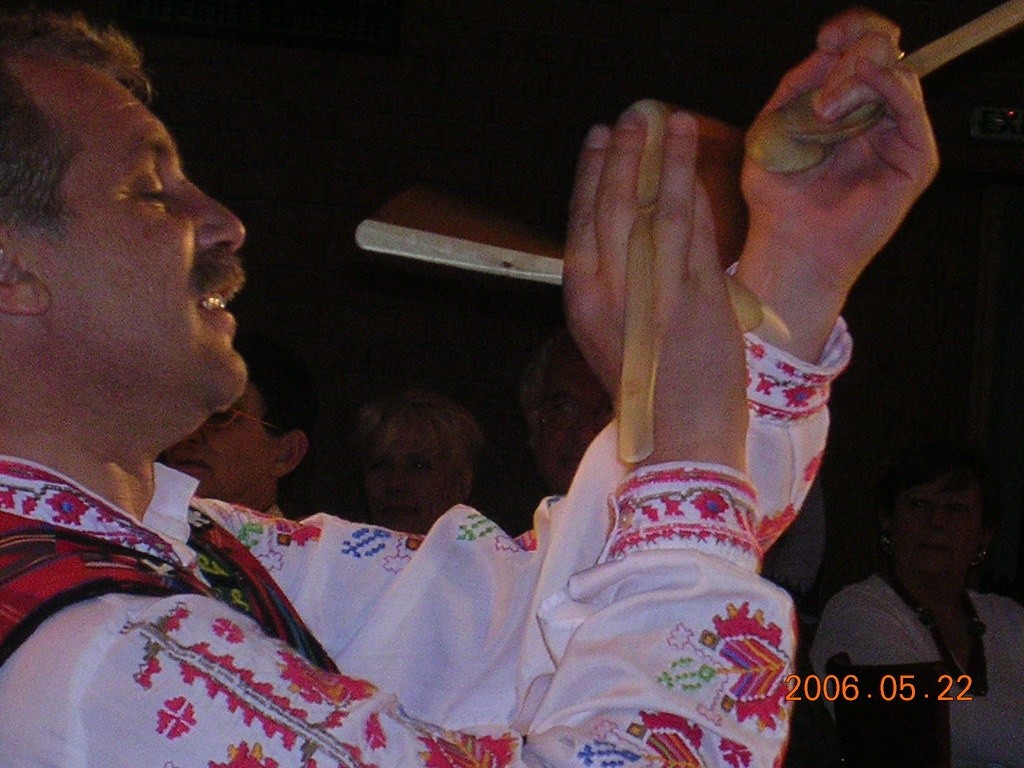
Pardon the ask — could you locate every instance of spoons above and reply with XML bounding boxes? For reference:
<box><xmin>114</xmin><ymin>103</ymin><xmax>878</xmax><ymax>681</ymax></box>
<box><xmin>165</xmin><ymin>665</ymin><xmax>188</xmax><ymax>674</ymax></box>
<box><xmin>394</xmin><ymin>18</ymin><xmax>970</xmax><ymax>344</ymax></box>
<box><xmin>356</xmin><ymin>217</ymin><xmax>765</xmax><ymax>333</ymax></box>
<box><xmin>781</xmin><ymin>1</ymin><xmax>1024</xmax><ymax>145</ymax></box>
<box><xmin>616</xmin><ymin>100</ymin><xmax>667</xmax><ymax>463</ymax></box>
<box><xmin>745</xmin><ymin>107</ymin><xmax>835</xmax><ymax>175</ymax></box>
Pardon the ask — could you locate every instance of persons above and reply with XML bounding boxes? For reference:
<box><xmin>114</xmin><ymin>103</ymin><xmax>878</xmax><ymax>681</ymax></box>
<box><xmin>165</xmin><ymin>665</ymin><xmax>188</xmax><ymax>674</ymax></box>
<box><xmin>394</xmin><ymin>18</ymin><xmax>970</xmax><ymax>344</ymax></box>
<box><xmin>1</xmin><ymin>10</ymin><xmax>1024</xmax><ymax>768</ymax></box>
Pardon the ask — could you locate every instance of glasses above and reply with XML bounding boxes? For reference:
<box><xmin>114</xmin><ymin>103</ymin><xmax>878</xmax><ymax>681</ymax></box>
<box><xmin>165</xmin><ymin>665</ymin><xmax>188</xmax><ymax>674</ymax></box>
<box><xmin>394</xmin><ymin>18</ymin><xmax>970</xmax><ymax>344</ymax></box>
<box><xmin>205</xmin><ymin>406</ymin><xmax>288</xmax><ymax>433</ymax></box>
